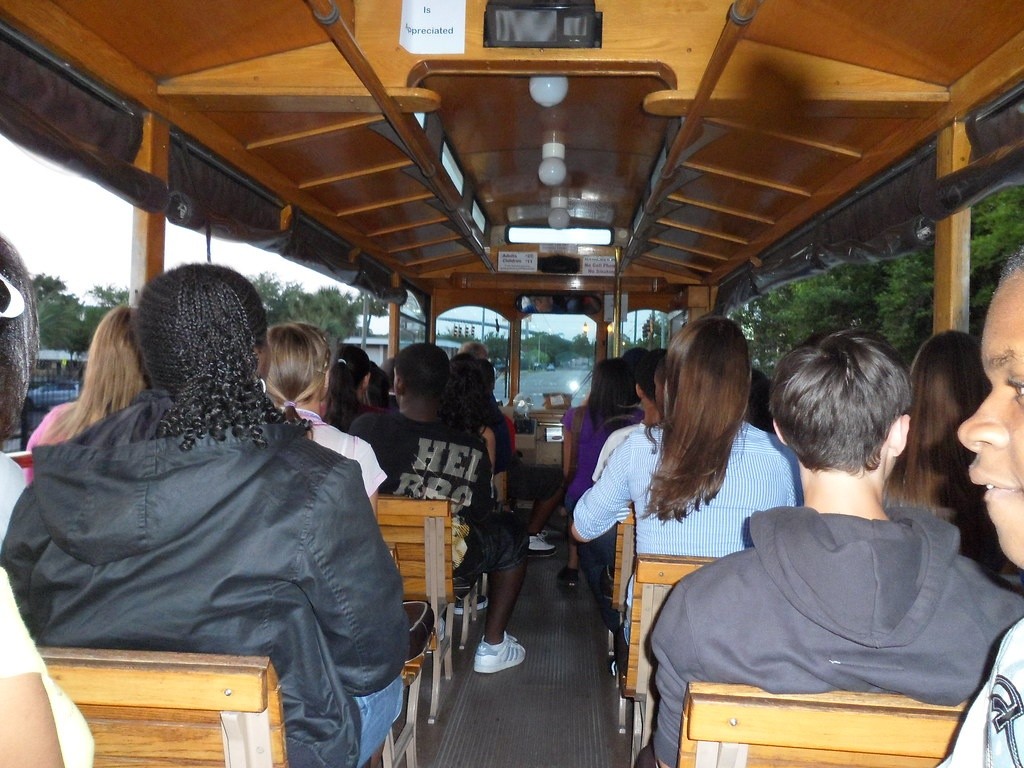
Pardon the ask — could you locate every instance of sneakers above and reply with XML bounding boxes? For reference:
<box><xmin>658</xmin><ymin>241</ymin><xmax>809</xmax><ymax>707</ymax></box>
<box><xmin>557</xmin><ymin>569</ymin><xmax>578</xmax><ymax>587</ymax></box>
<box><xmin>473</xmin><ymin>631</ymin><xmax>526</xmax><ymax>674</ymax></box>
<box><xmin>528</xmin><ymin>530</ymin><xmax>556</xmax><ymax>557</ymax></box>
<box><xmin>454</xmin><ymin>595</ymin><xmax>488</xmax><ymax>615</ymax></box>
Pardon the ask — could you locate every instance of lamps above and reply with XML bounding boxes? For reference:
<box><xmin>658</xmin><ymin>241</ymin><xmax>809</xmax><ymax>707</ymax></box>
<box><xmin>528</xmin><ymin>71</ymin><xmax>574</xmax><ymax>110</ymax></box>
<box><xmin>547</xmin><ymin>193</ymin><xmax>571</xmax><ymax>230</ymax></box>
<box><xmin>503</xmin><ymin>224</ymin><xmax>616</xmax><ymax>247</ymax></box>
<box><xmin>537</xmin><ymin>138</ymin><xmax>567</xmax><ymax>187</ymax></box>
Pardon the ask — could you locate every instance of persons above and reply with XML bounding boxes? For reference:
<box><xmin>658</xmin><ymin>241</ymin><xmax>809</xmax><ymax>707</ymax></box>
<box><xmin>933</xmin><ymin>251</ymin><xmax>1024</xmax><ymax>768</ymax></box>
<box><xmin>0</xmin><ymin>440</ymin><xmax>90</xmax><ymax>768</ymax></box>
<box><xmin>0</xmin><ymin>263</ymin><xmax>405</xmax><ymax>768</ymax></box>
<box><xmin>644</xmin><ymin>327</ymin><xmax>1024</xmax><ymax>768</ymax></box>
<box><xmin>264</xmin><ymin>322</ymin><xmax>389</xmax><ymax>534</ymax></box>
<box><xmin>28</xmin><ymin>306</ymin><xmax>153</xmax><ymax>478</ymax></box>
<box><xmin>560</xmin><ymin>310</ymin><xmax>1006</xmax><ymax>658</ymax></box>
<box><xmin>327</xmin><ymin>321</ymin><xmax>568</xmax><ymax>675</ymax></box>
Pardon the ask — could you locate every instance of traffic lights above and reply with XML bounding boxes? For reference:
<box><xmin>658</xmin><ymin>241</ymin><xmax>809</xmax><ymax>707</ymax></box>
<box><xmin>453</xmin><ymin>324</ymin><xmax>475</xmax><ymax>338</ymax></box>
<box><xmin>641</xmin><ymin>321</ymin><xmax>653</xmax><ymax>338</ymax></box>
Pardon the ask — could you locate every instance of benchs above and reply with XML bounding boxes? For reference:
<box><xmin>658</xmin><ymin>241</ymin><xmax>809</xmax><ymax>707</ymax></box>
<box><xmin>369</xmin><ymin>617</ymin><xmax>441</xmax><ymax>768</ymax></box>
<box><xmin>611</xmin><ymin>505</ymin><xmax>639</xmax><ymax>734</ymax></box>
<box><xmin>613</xmin><ymin>552</ymin><xmax>720</xmax><ymax>768</ymax></box>
<box><xmin>374</xmin><ymin>490</ymin><xmax>478</xmax><ymax>726</ymax></box>
<box><xmin>677</xmin><ymin>679</ymin><xmax>969</xmax><ymax>768</ymax></box>
<box><xmin>38</xmin><ymin>641</ymin><xmax>291</xmax><ymax>768</ymax></box>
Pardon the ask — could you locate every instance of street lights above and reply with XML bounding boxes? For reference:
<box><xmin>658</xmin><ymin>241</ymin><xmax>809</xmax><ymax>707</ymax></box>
<box><xmin>539</xmin><ymin>333</ymin><xmax>546</xmax><ymax>363</ymax></box>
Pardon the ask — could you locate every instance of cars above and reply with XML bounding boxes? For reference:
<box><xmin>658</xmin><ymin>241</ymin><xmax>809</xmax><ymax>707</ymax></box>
<box><xmin>24</xmin><ymin>381</ymin><xmax>79</xmax><ymax>411</ymax></box>
<box><xmin>495</xmin><ymin>362</ymin><xmax>509</xmax><ymax>372</ymax></box>
<box><xmin>547</xmin><ymin>364</ymin><xmax>555</xmax><ymax>370</ymax></box>
<box><xmin>532</xmin><ymin>363</ymin><xmax>542</xmax><ymax>370</ymax></box>
